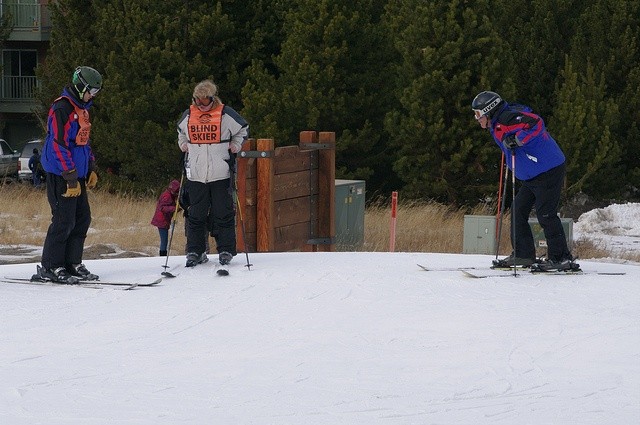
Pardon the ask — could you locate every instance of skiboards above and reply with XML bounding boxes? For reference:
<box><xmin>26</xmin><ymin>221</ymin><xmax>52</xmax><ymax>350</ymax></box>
<box><xmin>0</xmin><ymin>277</ymin><xmax>162</xmax><ymax>290</ymax></box>
<box><xmin>160</xmin><ymin>260</ymin><xmax>228</xmax><ymax>277</ymax></box>
<box><xmin>418</xmin><ymin>262</ymin><xmax>628</xmax><ymax>277</ymax></box>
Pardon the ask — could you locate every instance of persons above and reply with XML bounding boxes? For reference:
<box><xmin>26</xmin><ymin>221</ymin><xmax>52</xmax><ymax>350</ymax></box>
<box><xmin>471</xmin><ymin>90</ymin><xmax>573</xmax><ymax>271</ymax></box>
<box><xmin>176</xmin><ymin>80</ymin><xmax>249</xmax><ymax>266</ymax></box>
<box><xmin>29</xmin><ymin>148</ymin><xmax>41</xmax><ymax>186</ymax></box>
<box><xmin>38</xmin><ymin>65</ymin><xmax>103</xmax><ymax>284</ymax></box>
<box><xmin>151</xmin><ymin>179</ymin><xmax>180</xmax><ymax>256</ymax></box>
<box><xmin>179</xmin><ymin>177</ymin><xmax>210</xmax><ymax>254</ymax></box>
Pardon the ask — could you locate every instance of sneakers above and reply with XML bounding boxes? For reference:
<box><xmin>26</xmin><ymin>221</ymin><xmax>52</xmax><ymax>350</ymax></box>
<box><xmin>186</xmin><ymin>251</ymin><xmax>208</xmax><ymax>264</ymax></box>
<box><xmin>502</xmin><ymin>254</ymin><xmax>536</xmax><ymax>266</ymax></box>
<box><xmin>67</xmin><ymin>263</ymin><xmax>99</xmax><ymax>280</ymax></box>
<box><xmin>218</xmin><ymin>251</ymin><xmax>233</xmax><ymax>265</ymax></box>
<box><xmin>40</xmin><ymin>265</ymin><xmax>78</xmax><ymax>284</ymax></box>
<box><xmin>532</xmin><ymin>258</ymin><xmax>570</xmax><ymax>270</ymax></box>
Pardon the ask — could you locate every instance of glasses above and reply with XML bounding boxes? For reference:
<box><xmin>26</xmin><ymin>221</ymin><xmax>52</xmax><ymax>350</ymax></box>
<box><xmin>87</xmin><ymin>87</ymin><xmax>99</xmax><ymax>95</ymax></box>
<box><xmin>194</xmin><ymin>95</ymin><xmax>214</xmax><ymax>105</ymax></box>
<box><xmin>475</xmin><ymin>109</ymin><xmax>485</xmax><ymax>118</ymax></box>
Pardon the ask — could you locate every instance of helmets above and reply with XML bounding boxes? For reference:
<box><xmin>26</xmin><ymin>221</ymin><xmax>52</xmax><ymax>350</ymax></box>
<box><xmin>472</xmin><ymin>90</ymin><xmax>505</xmax><ymax>119</ymax></box>
<box><xmin>73</xmin><ymin>66</ymin><xmax>103</xmax><ymax>92</ymax></box>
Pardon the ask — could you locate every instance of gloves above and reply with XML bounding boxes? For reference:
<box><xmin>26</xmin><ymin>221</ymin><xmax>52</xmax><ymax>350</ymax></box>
<box><xmin>87</xmin><ymin>171</ymin><xmax>98</xmax><ymax>189</ymax></box>
<box><xmin>504</xmin><ymin>134</ymin><xmax>517</xmax><ymax>150</ymax></box>
<box><xmin>62</xmin><ymin>181</ymin><xmax>81</xmax><ymax>197</ymax></box>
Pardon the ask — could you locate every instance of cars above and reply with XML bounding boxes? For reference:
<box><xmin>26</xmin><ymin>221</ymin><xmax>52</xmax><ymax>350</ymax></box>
<box><xmin>17</xmin><ymin>139</ymin><xmax>43</xmax><ymax>183</ymax></box>
<box><xmin>0</xmin><ymin>139</ymin><xmax>21</xmax><ymax>178</ymax></box>
